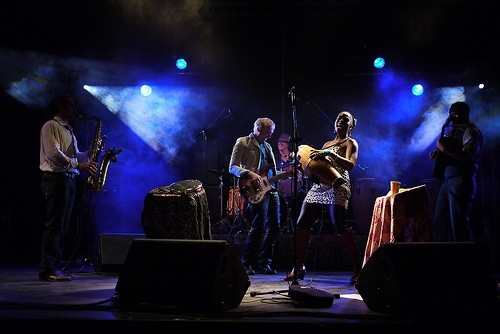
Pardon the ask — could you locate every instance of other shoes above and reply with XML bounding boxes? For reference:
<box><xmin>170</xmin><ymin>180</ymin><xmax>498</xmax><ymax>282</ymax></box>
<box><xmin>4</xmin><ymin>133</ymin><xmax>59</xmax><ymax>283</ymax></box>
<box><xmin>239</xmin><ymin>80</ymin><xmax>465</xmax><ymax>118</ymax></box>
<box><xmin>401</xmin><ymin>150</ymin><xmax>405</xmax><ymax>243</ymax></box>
<box><xmin>39</xmin><ymin>269</ymin><xmax>72</xmax><ymax>282</ymax></box>
<box><xmin>244</xmin><ymin>266</ymin><xmax>255</xmax><ymax>274</ymax></box>
<box><xmin>258</xmin><ymin>264</ymin><xmax>277</xmax><ymax>275</ymax></box>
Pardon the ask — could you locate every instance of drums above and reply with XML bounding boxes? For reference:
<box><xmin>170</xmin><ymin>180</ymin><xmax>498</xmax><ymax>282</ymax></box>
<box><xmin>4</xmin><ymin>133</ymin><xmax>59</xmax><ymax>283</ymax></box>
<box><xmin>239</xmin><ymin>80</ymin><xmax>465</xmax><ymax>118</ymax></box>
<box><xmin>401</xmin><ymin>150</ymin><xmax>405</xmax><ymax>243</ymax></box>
<box><xmin>291</xmin><ymin>143</ymin><xmax>345</xmax><ymax>187</ymax></box>
<box><xmin>226</xmin><ymin>186</ymin><xmax>246</xmax><ymax>217</ymax></box>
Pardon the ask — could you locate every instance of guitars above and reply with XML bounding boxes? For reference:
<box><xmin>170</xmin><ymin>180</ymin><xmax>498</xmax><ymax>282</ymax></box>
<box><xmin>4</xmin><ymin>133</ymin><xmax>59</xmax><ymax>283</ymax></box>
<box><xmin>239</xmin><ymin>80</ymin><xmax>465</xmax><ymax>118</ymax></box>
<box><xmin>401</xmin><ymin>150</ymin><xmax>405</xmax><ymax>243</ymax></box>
<box><xmin>238</xmin><ymin>167</ymin><xmax>300</xmax><ymax>204</ymax></box>
<box><xmin>433</xmin><ymin>112</ymin><xmax>462</xmax><ymax>180</ymax></box>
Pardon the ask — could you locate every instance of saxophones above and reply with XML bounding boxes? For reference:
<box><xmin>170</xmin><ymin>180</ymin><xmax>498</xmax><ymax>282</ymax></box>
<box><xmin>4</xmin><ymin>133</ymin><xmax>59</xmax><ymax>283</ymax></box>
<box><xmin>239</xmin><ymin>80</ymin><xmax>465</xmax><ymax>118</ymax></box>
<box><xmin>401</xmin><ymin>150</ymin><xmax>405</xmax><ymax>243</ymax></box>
<box><xmin>74</xmin><ymin>114</ymin><xmax>122</xmax><ymax>192</ymax></box>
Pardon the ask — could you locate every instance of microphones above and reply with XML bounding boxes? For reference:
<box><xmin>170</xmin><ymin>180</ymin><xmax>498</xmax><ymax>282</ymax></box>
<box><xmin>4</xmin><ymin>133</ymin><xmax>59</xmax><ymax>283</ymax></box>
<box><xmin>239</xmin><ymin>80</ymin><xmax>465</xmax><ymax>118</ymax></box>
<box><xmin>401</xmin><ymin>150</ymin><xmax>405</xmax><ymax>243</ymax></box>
<box><xmin>226</xmin><ymin>106</ymin><xmax>233</xmax><ymax>121</ymax></box>
<box><xmin>288</xmin><ymin>86</ymin><xmax>295</xmax><ymax>98</ymax></box>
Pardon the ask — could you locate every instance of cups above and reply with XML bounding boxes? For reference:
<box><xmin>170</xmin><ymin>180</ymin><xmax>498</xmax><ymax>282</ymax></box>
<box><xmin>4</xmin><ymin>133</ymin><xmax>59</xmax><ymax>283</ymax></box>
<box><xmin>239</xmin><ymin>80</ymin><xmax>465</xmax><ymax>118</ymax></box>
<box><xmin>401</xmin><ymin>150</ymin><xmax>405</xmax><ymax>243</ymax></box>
<box><xmin>390</xmin><ymin>181</ymin><xmax>401</xmax><ymax>195</ymax></box>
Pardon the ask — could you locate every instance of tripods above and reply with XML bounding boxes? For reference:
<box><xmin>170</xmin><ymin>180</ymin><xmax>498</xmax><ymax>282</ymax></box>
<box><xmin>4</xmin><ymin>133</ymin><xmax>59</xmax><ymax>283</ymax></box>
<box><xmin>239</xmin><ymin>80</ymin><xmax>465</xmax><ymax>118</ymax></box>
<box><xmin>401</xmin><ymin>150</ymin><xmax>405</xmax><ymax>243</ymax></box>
<box><xmin>249</xmin><ymin>91</ymin><xmax>341</xmax><ymax>300</ymax></box>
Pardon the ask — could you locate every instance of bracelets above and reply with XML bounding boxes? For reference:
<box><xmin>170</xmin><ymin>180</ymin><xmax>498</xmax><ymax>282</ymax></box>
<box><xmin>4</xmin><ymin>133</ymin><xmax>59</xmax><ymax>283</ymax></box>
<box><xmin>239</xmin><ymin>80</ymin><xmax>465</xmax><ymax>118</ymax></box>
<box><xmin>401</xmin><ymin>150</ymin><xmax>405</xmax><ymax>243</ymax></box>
<box><xmin>324</xmin><ymin>151</ymin><xmax>330</xmax><ymax>156</ymax></box>
<box><xmin>333</xmin><ymin>154</ymin><xmax>338</xmax><ymax>161</ymax></box>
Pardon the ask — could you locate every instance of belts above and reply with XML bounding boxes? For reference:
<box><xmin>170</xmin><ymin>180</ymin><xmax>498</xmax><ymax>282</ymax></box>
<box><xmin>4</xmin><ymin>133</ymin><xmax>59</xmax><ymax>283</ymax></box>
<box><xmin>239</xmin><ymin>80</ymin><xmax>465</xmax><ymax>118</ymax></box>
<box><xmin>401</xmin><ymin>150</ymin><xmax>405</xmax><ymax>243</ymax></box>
<box><xmin>43</xmin><ymin>171</ymin><xmax>75</xmax><ymax>179</ymax></box>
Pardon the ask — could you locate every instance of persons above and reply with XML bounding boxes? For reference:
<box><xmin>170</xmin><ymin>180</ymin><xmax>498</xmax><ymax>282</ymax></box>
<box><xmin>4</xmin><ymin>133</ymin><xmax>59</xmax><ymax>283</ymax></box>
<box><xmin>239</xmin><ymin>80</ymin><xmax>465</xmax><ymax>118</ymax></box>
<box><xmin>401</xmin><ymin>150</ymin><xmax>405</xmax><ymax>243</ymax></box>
<box><xmin>228</xmin><ymin>118</ymin><xmax>304</xmax><ymax>274</ymax></box>
<box><xmin>285</xmin><ymin>112</ymin><xmax>361</xmax><ymax>283</ymax></box>
<box><xmin>430</xmin><ymin>101</ymin><xmax>483</xmax><ymax>240</ymax></box>
<box><xmin>40</xmin><ymin>94</ymin><xmax>107</xmax><ymax>280</ymax></box>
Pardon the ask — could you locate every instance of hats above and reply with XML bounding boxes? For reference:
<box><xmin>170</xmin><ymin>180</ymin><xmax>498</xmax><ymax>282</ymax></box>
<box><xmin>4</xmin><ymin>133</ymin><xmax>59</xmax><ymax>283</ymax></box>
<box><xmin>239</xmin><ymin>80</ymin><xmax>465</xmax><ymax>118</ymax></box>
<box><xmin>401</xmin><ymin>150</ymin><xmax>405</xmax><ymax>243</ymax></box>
<box><xmin>275</xmin><ymin>133</ymin><xmax>292</xmax><ymax>144</ymax></box>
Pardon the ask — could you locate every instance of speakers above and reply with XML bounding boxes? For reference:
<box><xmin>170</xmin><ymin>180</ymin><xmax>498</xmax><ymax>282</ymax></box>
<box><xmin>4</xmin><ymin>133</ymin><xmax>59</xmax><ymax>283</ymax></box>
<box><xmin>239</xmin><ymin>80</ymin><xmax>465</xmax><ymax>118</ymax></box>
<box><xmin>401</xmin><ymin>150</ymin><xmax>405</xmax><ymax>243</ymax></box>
<box><xmin>92</xmin><ymin>233</ymin><xmax>146</xmax><ymax>275</ymax></box>
<box><xmin>115</xmin><ymin>238</ymin><xmax>251</xmax><ymax>311</ymax></box>
<box><xmin>354</xmin><ymin>240</ymin><xmax>496</xmax><ymax>315</ymax></box>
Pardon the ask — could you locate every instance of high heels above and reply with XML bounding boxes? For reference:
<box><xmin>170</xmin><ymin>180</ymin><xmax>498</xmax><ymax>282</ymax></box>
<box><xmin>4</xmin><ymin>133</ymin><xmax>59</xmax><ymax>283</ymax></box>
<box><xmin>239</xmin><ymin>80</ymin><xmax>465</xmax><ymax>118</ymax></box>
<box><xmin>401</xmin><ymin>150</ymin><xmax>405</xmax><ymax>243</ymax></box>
<box><xmin>350</xmin><ymin>272</ymin><xmax>359</xmax><ymax>286</ymax></box>
<box><xmin>284</xmin><ymin>267</ymin><xmax>306</xmax><ymax>281</ymax></box>
